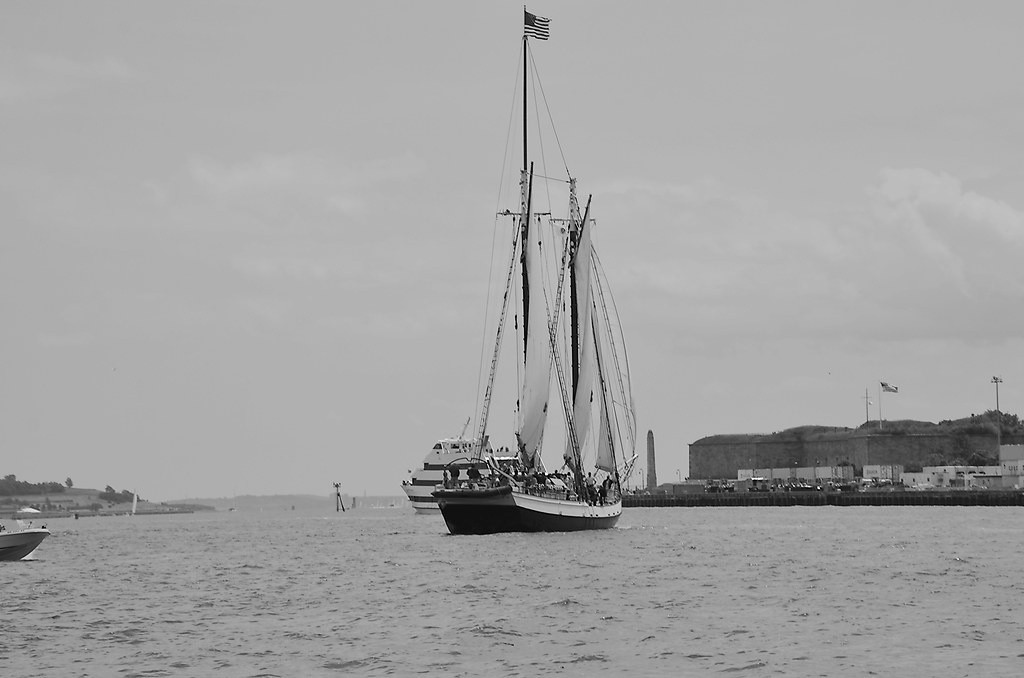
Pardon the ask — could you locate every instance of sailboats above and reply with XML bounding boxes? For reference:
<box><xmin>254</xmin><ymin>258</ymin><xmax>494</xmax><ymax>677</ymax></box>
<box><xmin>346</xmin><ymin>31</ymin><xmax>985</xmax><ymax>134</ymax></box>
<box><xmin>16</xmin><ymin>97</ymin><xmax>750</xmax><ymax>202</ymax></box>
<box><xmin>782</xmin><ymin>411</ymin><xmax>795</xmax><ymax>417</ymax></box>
<box><xmin>432</xmin><ymin>5</ymin><xmax>639</xmax><ymax>535</ymax></box>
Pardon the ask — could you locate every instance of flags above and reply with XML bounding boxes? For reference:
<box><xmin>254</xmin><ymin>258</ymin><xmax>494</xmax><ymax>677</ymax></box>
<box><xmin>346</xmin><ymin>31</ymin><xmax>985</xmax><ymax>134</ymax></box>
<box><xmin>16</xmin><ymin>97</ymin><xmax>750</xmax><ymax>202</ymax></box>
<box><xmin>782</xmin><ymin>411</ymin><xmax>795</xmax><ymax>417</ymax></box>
<box><xmin>880</xmin><ymin>382</ymin><xmax>899</xmax><ymax>393</ymax></box>
<box><xmin>524</xmin><ymin>10</ymin><xmax>552</xmax><ymax>40</ymax></box>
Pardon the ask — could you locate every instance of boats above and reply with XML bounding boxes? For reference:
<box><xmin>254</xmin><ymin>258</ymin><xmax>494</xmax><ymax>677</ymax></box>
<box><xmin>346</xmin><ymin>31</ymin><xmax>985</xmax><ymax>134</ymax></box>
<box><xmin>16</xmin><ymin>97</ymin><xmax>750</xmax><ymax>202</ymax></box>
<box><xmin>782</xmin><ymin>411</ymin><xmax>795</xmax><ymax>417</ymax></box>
<box><xmin>0</xmin><ymin>525</ymin><xmax>51</xmax><ymax>561</ymax></box>
<box><xmin>400</xmin><ymin>417</ymin><xmax>517</xmax><ymax>514</ymax></box>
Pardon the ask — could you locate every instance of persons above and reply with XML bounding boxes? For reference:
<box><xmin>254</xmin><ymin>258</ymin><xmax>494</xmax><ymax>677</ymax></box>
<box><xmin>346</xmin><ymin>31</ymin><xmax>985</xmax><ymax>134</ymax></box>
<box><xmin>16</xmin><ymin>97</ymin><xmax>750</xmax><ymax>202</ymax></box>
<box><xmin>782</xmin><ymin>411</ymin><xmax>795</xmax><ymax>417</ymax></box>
<box><xmin>530</xmin><ymin>469</ymin><xmax>573</xmax><ymax>485</ymax></box>
<box><xmin>449</xmin><ymin>462</ymin><xmax>460</xmax><ymax>481</ymax></box>
<box><xmin>598</xmin><ymin>475</ymin><xmax>613</xmax><ymax>500</ymax></box>
<box><xmin>467</xmin><ymin>464</ymin><xmax>475</xmax><ymax>480</ymax></box>
<box><xmin>583</xmin><ymin>472</ymin><xmax>597</xmax><ymax>496</ymax></box>
